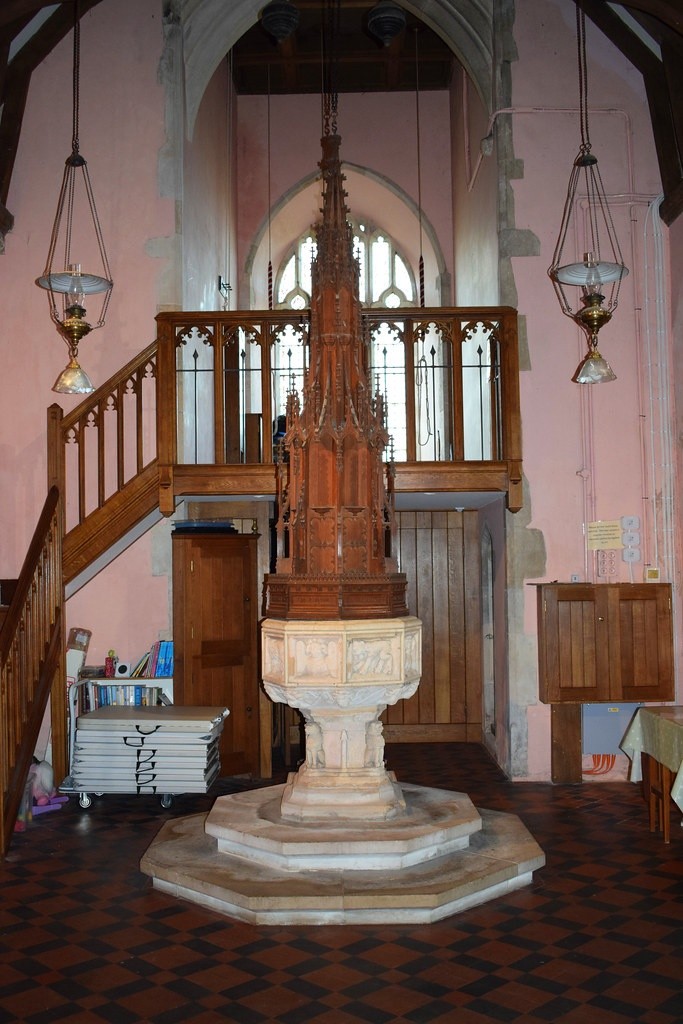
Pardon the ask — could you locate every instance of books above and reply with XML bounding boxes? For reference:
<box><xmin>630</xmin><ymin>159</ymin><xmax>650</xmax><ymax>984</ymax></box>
<box><xmin>78</xmin><ymin>684</ymin><xmax>172</xmax><ymax>717</ymax></box>
<box><xmin>131</xmin><ymin>640</ymin><xmax>174</xmax><ymax>677</ymax></box>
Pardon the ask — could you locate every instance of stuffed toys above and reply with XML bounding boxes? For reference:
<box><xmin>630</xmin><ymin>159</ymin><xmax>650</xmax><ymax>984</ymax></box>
<box><xmin>28</xmin><ymin>761</ymin><xmax>57</xmax><ymax>805</ymax></box>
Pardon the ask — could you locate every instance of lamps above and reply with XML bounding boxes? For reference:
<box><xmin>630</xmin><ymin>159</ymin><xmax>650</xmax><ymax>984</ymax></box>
<box><xmin>545</xmin><ymin>2</ymin><xmax>630</xmax><ymax>384</ymax></box>
<box><xmin>36</xmin><ymin>19</ymin><xmax>113</xmax><ymax>394</ymax></box>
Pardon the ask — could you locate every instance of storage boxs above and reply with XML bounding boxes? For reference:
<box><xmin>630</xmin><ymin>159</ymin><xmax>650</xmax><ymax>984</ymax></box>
<box><xmin>13</xmin><ymin>772</ymin><xmax>37</xmax><ymax>832</ymax></box>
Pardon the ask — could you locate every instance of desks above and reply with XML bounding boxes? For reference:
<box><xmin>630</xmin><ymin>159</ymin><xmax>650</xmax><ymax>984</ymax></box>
<box><xmin>620</xmin><ymin>705</ymin><xmax>683</xmax><ymax>844</ymax></box>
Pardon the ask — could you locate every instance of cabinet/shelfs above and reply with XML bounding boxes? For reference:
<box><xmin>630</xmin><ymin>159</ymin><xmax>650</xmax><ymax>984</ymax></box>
<box><xmin>537</xmin><ymin>583</ymin><xmax>674</xmax><ymax>704</ymax></box>
